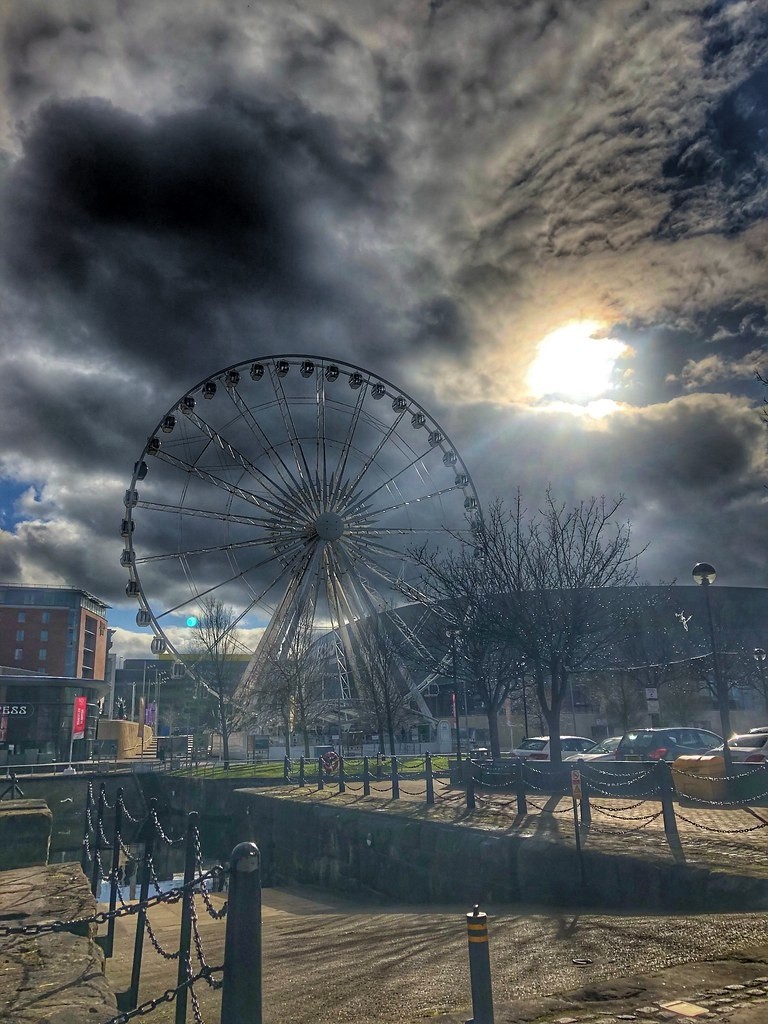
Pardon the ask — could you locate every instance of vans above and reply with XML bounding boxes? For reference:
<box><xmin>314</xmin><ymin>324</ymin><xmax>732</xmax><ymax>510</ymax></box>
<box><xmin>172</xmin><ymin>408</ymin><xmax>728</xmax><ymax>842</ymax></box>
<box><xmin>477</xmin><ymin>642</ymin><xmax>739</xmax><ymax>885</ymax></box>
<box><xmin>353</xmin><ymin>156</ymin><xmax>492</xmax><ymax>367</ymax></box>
<box><xmin>616</xmin><ymin>727</ymin><xmax>724</xmax><ymax>789</ymax></box>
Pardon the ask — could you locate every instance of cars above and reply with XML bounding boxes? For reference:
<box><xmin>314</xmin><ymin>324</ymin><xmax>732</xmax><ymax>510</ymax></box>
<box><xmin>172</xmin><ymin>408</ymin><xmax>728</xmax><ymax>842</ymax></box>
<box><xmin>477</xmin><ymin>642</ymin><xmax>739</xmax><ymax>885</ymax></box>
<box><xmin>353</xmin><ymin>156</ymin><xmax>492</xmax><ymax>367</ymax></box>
<box><xmin>704</xmin><ymin>727</ymin><xmax>768</xmax><ymax>789</ymax></box>
<box><xmin>193</xmin><ymin>748</ymin><xmax>210</xmax><ymax>760</ymax></box>
<box><xmin>561</xmin><ymin>736</ymin><xmax>651</xmax><ymax>791</ymax></box>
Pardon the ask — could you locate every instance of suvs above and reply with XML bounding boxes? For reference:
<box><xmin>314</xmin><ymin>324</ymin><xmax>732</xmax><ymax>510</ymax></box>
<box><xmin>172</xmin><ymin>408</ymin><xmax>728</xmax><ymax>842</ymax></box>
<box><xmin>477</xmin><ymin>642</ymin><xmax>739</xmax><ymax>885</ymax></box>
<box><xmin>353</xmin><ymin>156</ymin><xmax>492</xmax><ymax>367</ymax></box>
<box><xmin>511</xmin><ymin>736</ymin><xmax>613</xmax><ymax>781</ymax></box>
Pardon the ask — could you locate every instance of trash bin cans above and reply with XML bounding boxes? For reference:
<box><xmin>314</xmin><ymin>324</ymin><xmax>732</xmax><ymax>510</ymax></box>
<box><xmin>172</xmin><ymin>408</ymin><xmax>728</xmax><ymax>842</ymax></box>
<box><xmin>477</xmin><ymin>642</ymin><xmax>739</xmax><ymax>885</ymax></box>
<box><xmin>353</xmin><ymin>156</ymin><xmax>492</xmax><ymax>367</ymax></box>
<box><xmin>469</xmin><ymin>747</ymin><xmax>493</xmax><ymax>789</ymax></box>
<box><xmin>667</xmin><ymin>754</ymin><xmax>728</xmax><ymax>805</ymax></box>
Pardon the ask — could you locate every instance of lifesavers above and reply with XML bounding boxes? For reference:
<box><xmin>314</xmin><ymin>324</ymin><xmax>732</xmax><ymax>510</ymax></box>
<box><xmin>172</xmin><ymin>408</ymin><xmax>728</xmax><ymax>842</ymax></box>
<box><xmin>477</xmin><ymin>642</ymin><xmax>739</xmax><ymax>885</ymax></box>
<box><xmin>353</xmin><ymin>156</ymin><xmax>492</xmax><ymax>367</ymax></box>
<box><xmin>323</xmin><ymin>752</ymin><xmax>339</xmax><ymax>770</ymax></box>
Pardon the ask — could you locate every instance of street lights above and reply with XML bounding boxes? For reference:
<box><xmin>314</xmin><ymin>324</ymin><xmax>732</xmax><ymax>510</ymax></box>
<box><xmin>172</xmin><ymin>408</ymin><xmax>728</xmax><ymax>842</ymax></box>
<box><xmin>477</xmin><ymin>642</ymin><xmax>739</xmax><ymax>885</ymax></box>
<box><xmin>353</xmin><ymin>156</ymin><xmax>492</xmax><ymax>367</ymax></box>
<box><xmin>752</xmin><ymin>648</ymin><xmax>768</xmax><ymax>716</ymax></box>
<box><xmin>446</xmin><ymin>623</ymin><xmax>464</xmax><ymax>784</ymax></box>
<box><xmin>692</xmin><ymin>563</ymin><xmax>740</xmax><ymax>801</ymax></box>
